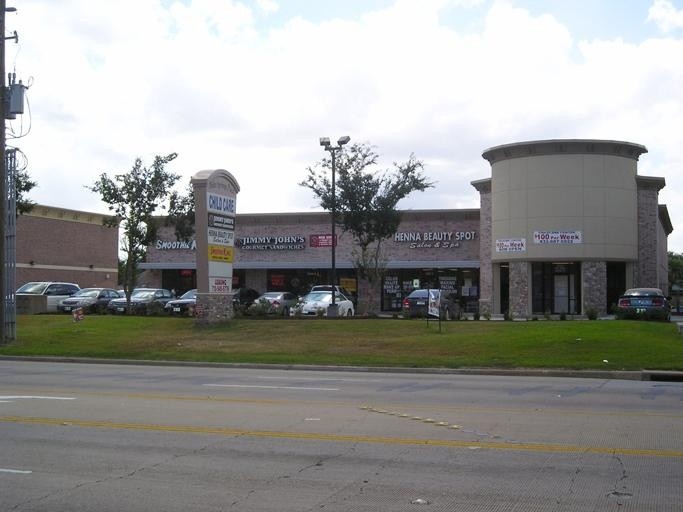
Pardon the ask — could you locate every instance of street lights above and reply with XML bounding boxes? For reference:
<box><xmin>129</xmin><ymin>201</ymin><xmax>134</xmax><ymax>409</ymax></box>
<box><xmin>320</xmin><ymin>136</ymin><xmax>351</xmax><ymax>317</ymax></box>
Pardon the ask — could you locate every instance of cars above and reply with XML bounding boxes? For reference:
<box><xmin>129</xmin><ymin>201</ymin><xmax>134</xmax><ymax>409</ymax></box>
<box><xmin>233</xmin><ymin>285</ymin><xmax>355</xmax><ymax>317</ymax></box>
<box><xmin>5</xmin><ymin>282</ymin><xmax>197</xmax><ymax>316</ymax></box>
<box><xmin>401</xmin><ymin>288</ymin><xmax>464</xmax><ymax>320</ymax></box>
<box><xmin>616</xmin><ymin>288</ymin><xmax>670</xmax><ymax>321</ymax></box>
